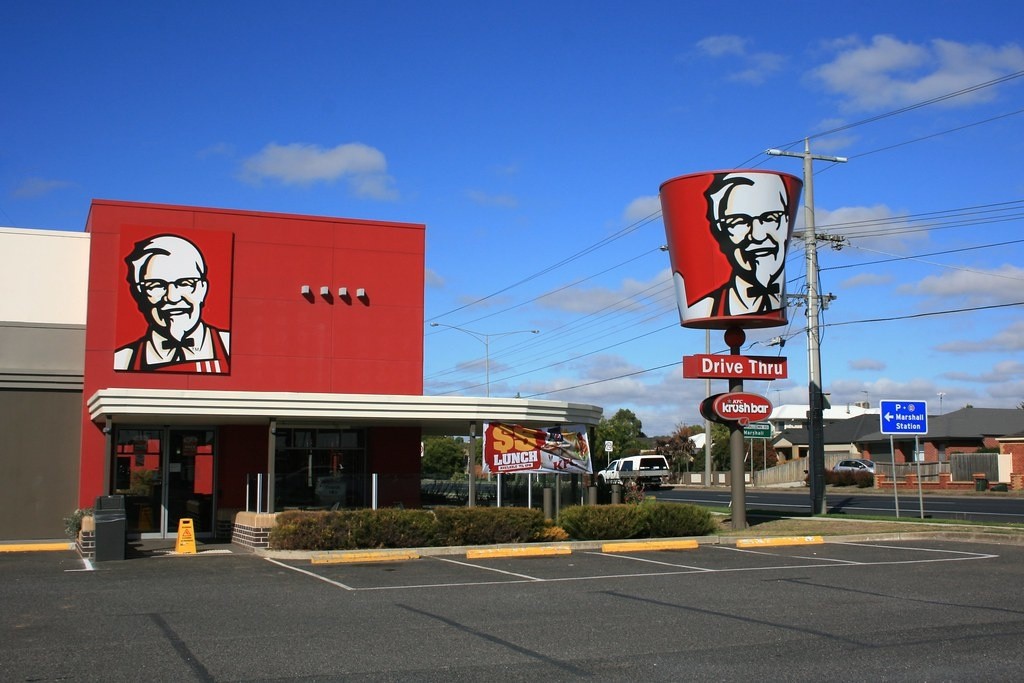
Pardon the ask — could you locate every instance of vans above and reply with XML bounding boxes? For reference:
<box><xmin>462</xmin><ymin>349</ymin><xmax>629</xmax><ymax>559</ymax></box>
<box><xmin>597</xmin><ymin>454</ymin><xmax>673</xmax><ymax>492</ymax></box>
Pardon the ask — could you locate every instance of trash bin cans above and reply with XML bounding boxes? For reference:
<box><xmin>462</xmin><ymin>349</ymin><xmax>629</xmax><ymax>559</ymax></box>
<box><xmin>976</xmin><ymin>478</ymin><xmax>988</xmax><ymax>491</ymax></box>
<box><xmin>94</xmin><ymin>495</ymin><xmax>126</xmax><ymax>563</ymax></box>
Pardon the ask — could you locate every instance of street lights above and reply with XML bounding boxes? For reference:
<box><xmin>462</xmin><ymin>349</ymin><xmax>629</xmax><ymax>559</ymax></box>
<box><xmin>430</xmin><ymin>321</ymin><xmax>541</xmax><ymax>397</ymax></box>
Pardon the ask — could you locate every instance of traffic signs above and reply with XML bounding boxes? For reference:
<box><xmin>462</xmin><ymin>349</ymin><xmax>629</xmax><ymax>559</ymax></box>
<box><xmin>879</xmin><ymin>400</ymin><xmax>928</xmax><ymax>435</ymax></box>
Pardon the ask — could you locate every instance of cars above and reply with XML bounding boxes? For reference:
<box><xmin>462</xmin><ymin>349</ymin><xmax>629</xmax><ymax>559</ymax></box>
<box><xmin>832</xmin><ymin>458</ymin><xmax>877</xmax><ymax>476</ymax></box>
